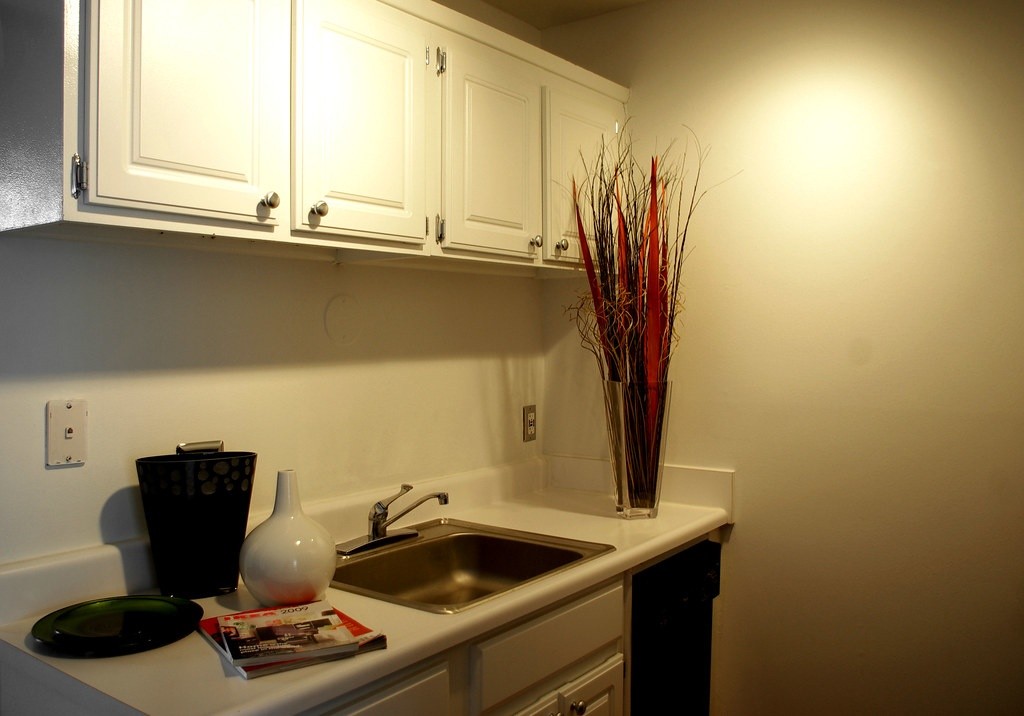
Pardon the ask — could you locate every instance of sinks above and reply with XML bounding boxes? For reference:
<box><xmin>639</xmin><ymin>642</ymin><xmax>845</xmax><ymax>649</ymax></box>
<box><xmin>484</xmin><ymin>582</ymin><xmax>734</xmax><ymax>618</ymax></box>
<box><xmin>328</xmin><ymin>517</ymin><xmax>617</xmax><ymax>616</ymax></box>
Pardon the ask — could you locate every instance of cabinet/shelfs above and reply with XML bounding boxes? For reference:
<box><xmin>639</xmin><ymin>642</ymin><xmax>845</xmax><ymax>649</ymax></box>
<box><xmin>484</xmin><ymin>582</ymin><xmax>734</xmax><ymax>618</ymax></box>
<box><xmin>0</xmin><ymin>0</ymin><xmax>290</xmax><ymax>256</ymax></box>
<box><xmin>542</xmin><ymin>66</ymin><xmax>629</xmax><ymax>274</ymax></box>
<box><xmin>472</xmin><ymin>572</ymin><xmax>626</xmax><ymax>716</ymax></box>
<box><xmin>290</xmin><ymin>0</ymin><xmax>430</xmax><ymax>267</ymax></box>
<box><xmin>430</xmin><ymin>22</ymin><xmax>543</xmax><ymax>277</ymax></box>
<box><xmin>332</xmin><ymin>660</ymin><xmax>451</xmax><ymax>716</ymax></box>
<box><xmin>631</xmin><ymin>538</ymin><xmax>721</xmax><ymax>716</ymax></box>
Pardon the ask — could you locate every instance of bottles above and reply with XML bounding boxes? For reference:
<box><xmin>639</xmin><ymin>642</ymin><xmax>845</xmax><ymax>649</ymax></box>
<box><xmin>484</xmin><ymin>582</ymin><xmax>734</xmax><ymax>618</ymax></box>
<box><xmin>239</xmin><ymin>469</ymin><xmax>337</xmax><ymax>610</ymax></box>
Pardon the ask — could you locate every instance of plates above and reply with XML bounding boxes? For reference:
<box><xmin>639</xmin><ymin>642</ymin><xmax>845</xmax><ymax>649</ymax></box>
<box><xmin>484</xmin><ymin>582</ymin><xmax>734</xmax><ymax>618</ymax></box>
<box><xmin>31</xmin><ymin>594</ymin><xmax>204</xmax><ymax>655</ymax></box>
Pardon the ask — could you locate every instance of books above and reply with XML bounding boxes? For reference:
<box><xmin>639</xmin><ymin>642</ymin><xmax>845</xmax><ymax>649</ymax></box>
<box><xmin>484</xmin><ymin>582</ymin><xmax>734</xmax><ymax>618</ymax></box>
<box><xmin>199</xmin><ymin>600</ymin><xmax>387</xmax><ymax>680</ymax></box>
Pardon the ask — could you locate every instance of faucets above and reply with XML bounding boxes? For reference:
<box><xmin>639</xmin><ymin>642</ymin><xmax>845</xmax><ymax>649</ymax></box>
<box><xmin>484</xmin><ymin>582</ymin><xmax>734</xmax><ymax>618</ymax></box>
<box><xmin>367</xmin><ymin>483</ymin><xmax>449</xmax><ymax>538</ymax></box>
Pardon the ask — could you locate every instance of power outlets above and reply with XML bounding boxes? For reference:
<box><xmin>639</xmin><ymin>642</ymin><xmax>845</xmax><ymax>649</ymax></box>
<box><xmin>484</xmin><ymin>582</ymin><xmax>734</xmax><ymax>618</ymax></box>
<box><xmin>523</xmin><ymin>404</ymin><xmax>536</xmax><ymax>441</ymax></box>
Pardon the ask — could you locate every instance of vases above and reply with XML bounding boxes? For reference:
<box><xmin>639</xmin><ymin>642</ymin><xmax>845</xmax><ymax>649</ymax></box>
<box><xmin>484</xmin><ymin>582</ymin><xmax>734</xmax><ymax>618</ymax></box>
<box><xmin>602</xmin><ymin>379</ymin><xmax>673</xmax><ymax>519</ymax></box>
<box><xmin>240</xmin><ymin>469</ymin><xmax>334</xmax><ymax>606</ymax></box>
